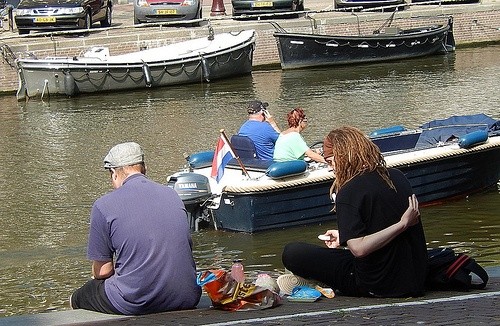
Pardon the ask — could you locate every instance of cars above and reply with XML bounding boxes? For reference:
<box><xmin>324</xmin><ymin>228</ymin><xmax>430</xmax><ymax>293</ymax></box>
<box><xmin>13</xmin><ymin>0</ymin><xmax>112</xmax><ymax>36</ymax></box>
<box><xmin>133</xmin><ymin>0</ymin><xmax>205</xmax><ymax>28</ymax></box>
<box><xmin>231</xmin><ymin>0</ymin><xmax>480</xmax><ymax>22</ymax></box>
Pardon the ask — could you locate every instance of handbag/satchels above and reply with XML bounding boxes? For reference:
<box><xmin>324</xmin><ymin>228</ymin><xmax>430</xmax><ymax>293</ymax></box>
<box><xmin>196</xmin><ymin>269</ymin><xmax>282</xmax><ymax>311</ymax></box>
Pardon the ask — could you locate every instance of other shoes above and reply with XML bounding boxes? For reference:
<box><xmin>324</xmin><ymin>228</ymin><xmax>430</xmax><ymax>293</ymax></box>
<box><xmin>277</xmin><ymin>274</ymin><xmax>336</xmax><ymax>298</ymax></box>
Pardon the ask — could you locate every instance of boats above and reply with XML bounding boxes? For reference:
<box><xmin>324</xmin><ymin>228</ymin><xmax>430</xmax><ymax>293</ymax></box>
<box><xmin>273</xmin><ymin>15</ymin><xmax>457</xmax><ymax>72</ymax></box>
<box><xmin>13</xmin><ymin>29</ymin><xmax>257</xmax><ymax>103</ymax></box>
<box><xmin>163</xmin><ymin>113</ymin><xmax>499</xmax><ymax>234</ymax></box>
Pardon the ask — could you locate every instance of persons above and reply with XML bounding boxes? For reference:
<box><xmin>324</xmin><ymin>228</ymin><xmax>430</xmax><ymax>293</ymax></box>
<box><xmin>273</xmin><ymin>107</ymin><xmax>328</xmax><ymax>164</ymax></box>
<box><xmin>237</xmin><ymin>100</ymin><xmax>281</xmax><ymax>160</ymax></box>
<box><xmin>282</xmin><ymin>125</ymin><xmax>432</xmax><ymax>299</ymax></box>
<box><xmin>70</xmin><ymin>141</ymin><xmax>202</xmax><ymax>316</ymax></box>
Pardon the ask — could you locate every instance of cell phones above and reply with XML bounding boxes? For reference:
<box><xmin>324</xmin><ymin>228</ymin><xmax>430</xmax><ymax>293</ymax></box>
<box><xmin>262</xmin><ymin>109</ymin><xmax>267</xmax><ymax>117</ymax></box>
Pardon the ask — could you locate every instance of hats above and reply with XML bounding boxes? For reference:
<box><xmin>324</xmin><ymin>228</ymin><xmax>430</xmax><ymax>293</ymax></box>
<box><xmin>446</xmin><ymin>253</ymin><xmax>489</xmax><ymax>289</ymax></box>
<box><xmin>246</xmin><ymin>100</ymin><xmax>269</xmax><ymax>114</ymax></box>
<box><xmin>103</xmin><ymin>142</ymin><xmax>145</xmax><ymax>169</ymax></box>
